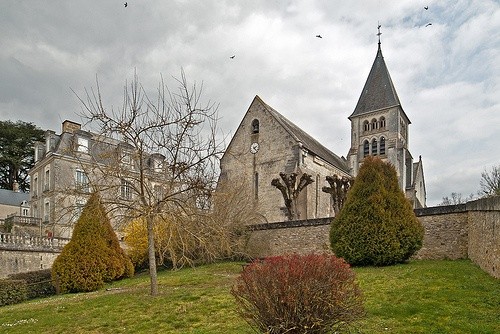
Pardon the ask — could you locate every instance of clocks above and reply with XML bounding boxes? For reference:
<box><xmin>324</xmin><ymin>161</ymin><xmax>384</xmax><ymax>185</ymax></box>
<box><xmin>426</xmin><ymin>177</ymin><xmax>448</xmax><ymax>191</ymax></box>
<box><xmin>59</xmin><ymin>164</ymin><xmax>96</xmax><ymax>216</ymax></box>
<box><xmin>250</xmin><ymin>142</ymin><xmax>260</xmax><ymax>154</ymax></box>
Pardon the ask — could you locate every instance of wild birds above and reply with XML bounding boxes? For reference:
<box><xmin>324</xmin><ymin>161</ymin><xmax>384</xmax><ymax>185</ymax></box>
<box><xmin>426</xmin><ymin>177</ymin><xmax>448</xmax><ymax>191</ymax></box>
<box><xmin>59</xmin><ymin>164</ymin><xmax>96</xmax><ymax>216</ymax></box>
<box><xmin>124</xmin><ymin>2</ymin><xmax>128</xmax><ymax>7</ymax></box>
<box><xmin>424</xmin><ymin>5</ymin><xmax>429</xmax><ymax>10</ymax></box>
<box><xmin>316</xmin><ymin>35</ymin><xmax>322</xmax><ymax>38</ymax></box>
<box><xmin>425</xmin><ymin>23</ymin><xmax>432</xmax><ymax>27</ymax></box>
<box><xmin>230</xmin><ymin>56</ymin><xmax>235</xmax><ymax>58</ymax></box>
